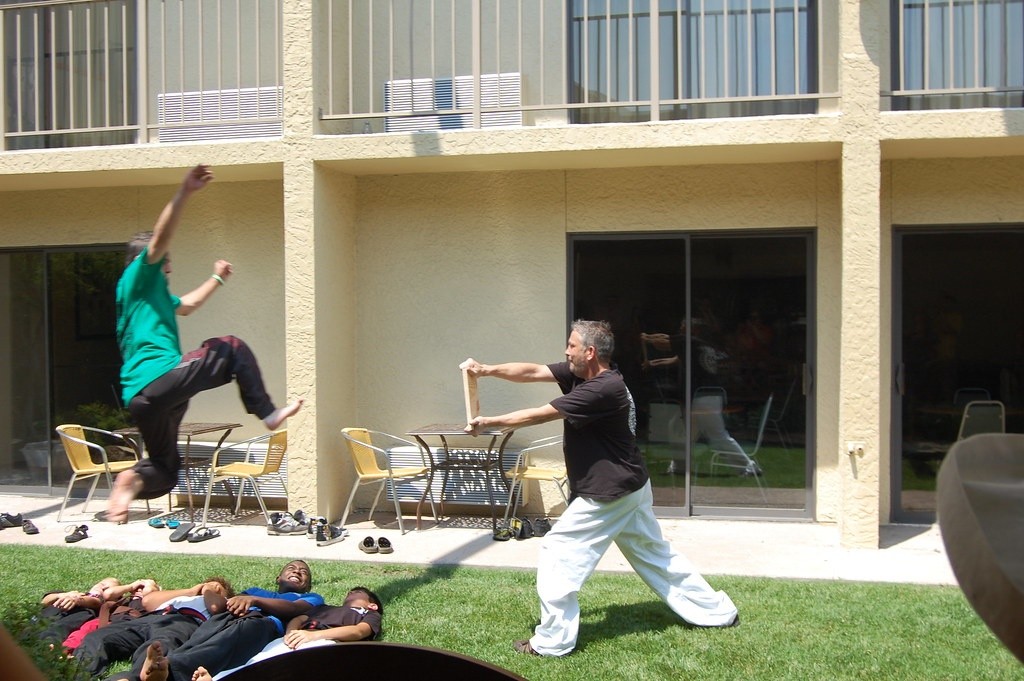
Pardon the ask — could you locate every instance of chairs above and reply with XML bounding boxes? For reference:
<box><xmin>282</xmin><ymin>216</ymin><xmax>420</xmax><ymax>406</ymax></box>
<box><xmin>55</xmin><ymin>423</ymin><xmax>151</xmax><ymax>523</ymax></box>
<box><xmin>501</xmin><ymin>434</ymin><xmax>571</xmax><ymax>532</ymax></box>
<box><xmin>338</xmin><ymin>426</ymin><xmax>440</xmax><ymax>536</ymax></box>
<box><xmin>202</xmin><ymin>428</ymin><xmax>289</xmax><ymax>529</ymax></box>
<box><xmin>639</xmin><ymin>365</ymin><xmax>803</xmax><ymax>507</ymax></box>
<box><xmin>897</xmin><ymin>385</ymin><xmax>1022</xmax><ymax>456</ymax></box>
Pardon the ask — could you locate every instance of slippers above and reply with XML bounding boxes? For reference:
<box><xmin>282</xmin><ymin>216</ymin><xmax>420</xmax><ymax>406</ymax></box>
<box><xmin>166</xmin><ymin>518</ymin><xmax>180</xmax><ymax>529</ymax></box>
<box><xmin>188</xmin><ymin>527</ymin><xmax>220</xmax><ymax>543</ymax></box>
<box><xmin>375</xmin><ymin>537</ymin><xmax>393</xmax><ymax>554</ymax></box>
<box><xmin>169</xmin><ymin>523</ymin><xmax>195</xmax><ymax>542</ymax></box>
<box><xmin>359</xmin><ymin>536</ymin><xmax>378</xmax><ymax>553</ymax></box>
<box><xmin>148</xmin><ymin>518</ymin><xmax>163</xmax><ymax>528</ymax></box>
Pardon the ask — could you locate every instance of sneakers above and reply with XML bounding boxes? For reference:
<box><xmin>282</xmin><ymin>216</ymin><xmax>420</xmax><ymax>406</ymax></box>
<box><xmin>316</xmin><ymin>523</ymin><xmax>344</xmax><ymax>547</ymax></box>
<box><xmin>267</xmin><ymin>512</ymin><xmax>291</xmax><ymax>535</ymax></box>
<box><xmin>742</xmin><ymin>461</ymin><xmax>760</xmax><ymax>477</ymax></box>
<box><xmin>278</xmin><ymin>511</ymin><xmax>308</xmax><ymax>535</ymax></box>
<box><xmin>65</xmin><ymin>525</ymin><xmax>89</xmax><ymax>543</ymax></box>
<box><xmin>533</xmin><ymin>517</ymin><xmax>551</xmax><ymax>537</ymax></box>
<box><xmin>510</xmin><ymin>516</ymin><xmax>522</xmax><ymax>539</ymax></box>
<box><xmin>663</xmin><ymin>461</ymin><xmax>684</xmax><ymax>474</ymax></box>
<box><xmin>0</xmin><ymin>513</ymin><xmax>23</xmax><ymax>527</ymax></box>
<box><xmin>307</xmin><ymin>516</ymin><xmax>347</xmax><ymax>538</ymax></box>
<box><xmin>520</xmin><ymin>517</ymin><xmax>534</xmax><ymax>537</ymax></box>
<box><xmin>23</xmin><ymin>519</ymin><xmax>38</xmax><ymax>534</ymax></box>
<box><xmin>492</xmin><ymin>527</ymin><xmax>511</xmax><ymax>540</ymax></box>
<box><xmin>513</xmin><ymin>639</ymin><xmax>540</xmax><ymax>655</ymax></box>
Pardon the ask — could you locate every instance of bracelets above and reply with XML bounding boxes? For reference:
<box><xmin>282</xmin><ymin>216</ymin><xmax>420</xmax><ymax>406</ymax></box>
<box><xmin>212</xmin><ymin>273</ymin><xmax>224</xmax><ymax>285</ymax></box>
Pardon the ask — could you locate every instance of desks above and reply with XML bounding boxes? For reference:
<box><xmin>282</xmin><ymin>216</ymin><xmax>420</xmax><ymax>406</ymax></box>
<box><xmin>111</xmin><ymin>422</ymin><xmax>245</xmax><ymax>523</ymax></box>
<box><xmin>404</xmin><ymin>422</ymin><xmax>525</xmax><ymax>533</ymax></box>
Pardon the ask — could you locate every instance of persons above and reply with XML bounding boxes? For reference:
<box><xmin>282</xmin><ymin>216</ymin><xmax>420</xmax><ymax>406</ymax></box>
<box><xmin>103</xmin><ymin>165</ymin><xmax>303</xmax><ymax>526</ymax></box>
<box><xmin>641</xmin><ymin>318</ymin><xmax>761</xmax><ymax>481</ymax></box>
<box><xmin>0</xmin><ymin>559</ymin><xmax>382</xmax><ymax>681</ymax></box>
<box><xmin>458</xmin><ymin>320</ymin><xmax>740</xmax><ymax>658</ymax></box>
